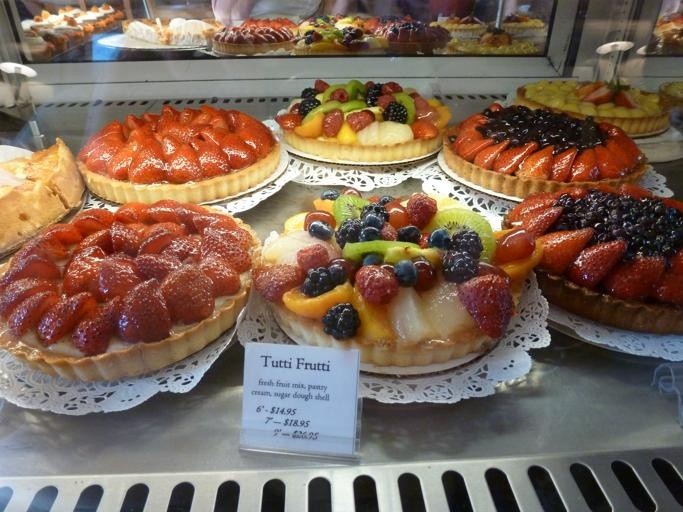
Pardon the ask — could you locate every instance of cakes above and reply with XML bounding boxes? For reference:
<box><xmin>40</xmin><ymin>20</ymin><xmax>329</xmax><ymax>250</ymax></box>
<box><xmin>76</xmin><ymin>104</ymin><xmax>281</xmax><ymax>205</ymax></box>
<box><xmin>502</xmin><ymin>183</ymin><xmax>683</xmax><ymax>335</ymax></box>
<box><xmin>645</xmin><ymin>11</ymin><xmax>683</xmax><ymax>56</ymax></box>
<box><xmin>506</xmin><ymin>79</ymin><xmax>672</xmax><ymax>138</ymax></box>
<box><xmin>21</xmin><ymin>3</ymin><xmax>546</xmax><ymax>56</ymax></box>
<box><xmin>252</xmin><ymin>187</ymin><xmax>543</xmax><ymax>367</ymax></box>
<box><xmin>275</xmin><ymin>79</ymin><xmax>452</xmax><ymax>162</ymax></box>
<box><xmin>659</xmin><ymin>82</ymin><xmax>683</xmax><ymax>108</ymax></box>
<box><xmin>0</xmin><ymin>200</ymin><xmax>263</xmax><ymax>380</ymax></box>
<box><xmin>0</xmin><ymin>137</ymin><xmax>87</xmax><ymax>259</ymax></box>
<box><xmin>443</xmin><ymin>103</ymin><xmax>649</xmax><ymax>199</ymax></box>
